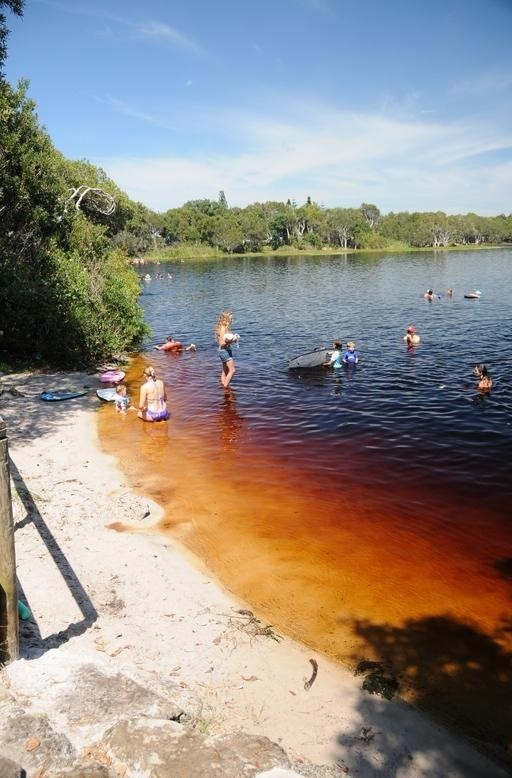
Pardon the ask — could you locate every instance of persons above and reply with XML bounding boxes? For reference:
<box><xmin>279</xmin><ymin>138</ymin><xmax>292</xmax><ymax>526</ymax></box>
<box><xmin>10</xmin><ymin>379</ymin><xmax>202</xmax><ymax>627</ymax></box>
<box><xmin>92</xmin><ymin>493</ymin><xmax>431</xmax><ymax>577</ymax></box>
<box><xmin>215</xmin><ymin>310</ymin><xmax>240</xmax><ymax>392</ymax></box>
<box><xmin>136</xmin><ymin>365</ymin><xmax>170</xmax><ymax>422</ymax></box>
<box><xmin>474</xmin><ymin>363</ymin><xmax>492</xmax><ymax>388</ymax></box>
<box><xmin>342</xmin><ymin>341</ymin><xmax>359</xmax><ymax>365</ymax></box>
<box><xmin>423</xmin><ymin>289</ymin><xmax>437</xmax><ymax>300</ymax></box>
<box><xmin>322</xmin><ymin>339</ymin><xmax>344</xmax><ymax>368</ymax></box>
<box><xmin>403</xmin><ymin>325</ymin><xmax>420</xmax><ymax>345</ymax></box>
<box><xmin>154</xmin><ymin>335</ymin><xmax>197</xmax><ymax>354</ymax></box>
<box><xmin>115</xmin><ymin>384</ymin><xmax>139</xmax><ymax>417</ymax></box>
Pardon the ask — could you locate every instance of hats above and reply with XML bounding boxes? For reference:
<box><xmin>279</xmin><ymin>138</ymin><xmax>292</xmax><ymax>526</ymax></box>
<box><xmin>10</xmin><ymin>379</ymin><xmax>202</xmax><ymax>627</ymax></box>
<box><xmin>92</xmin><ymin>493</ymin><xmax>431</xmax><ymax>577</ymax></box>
<box><xmin>406</xmin><ymin>326</ymin><xmax>416</xmax><ymax>332</ymax></box>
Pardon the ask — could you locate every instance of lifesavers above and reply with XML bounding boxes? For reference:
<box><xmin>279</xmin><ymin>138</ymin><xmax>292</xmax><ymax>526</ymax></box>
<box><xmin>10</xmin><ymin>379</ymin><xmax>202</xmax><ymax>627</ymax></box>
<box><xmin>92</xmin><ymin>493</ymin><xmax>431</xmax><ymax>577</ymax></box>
<box><xmin>164</xmin><ymin>342</ymin><xmax>182</xmax><ymax>351</ymax></box>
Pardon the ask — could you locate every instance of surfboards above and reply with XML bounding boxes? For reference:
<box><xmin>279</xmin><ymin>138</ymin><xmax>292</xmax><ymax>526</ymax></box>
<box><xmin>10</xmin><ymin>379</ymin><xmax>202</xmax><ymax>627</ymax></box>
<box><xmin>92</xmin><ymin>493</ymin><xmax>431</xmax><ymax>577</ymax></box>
<box><xmin>464</xmin><ymin>294</ymin><xmax>479</xmax><ymax>298</ymax></box>
<box><xmin>40</xmin><ymin>388</ymin><xmax>88</xmax><ymax>401</ymax></box>
<box><xmin>100</xmin><ymin>371</ymin><xmax>125</xmax><ymax>382</ymax></box>
